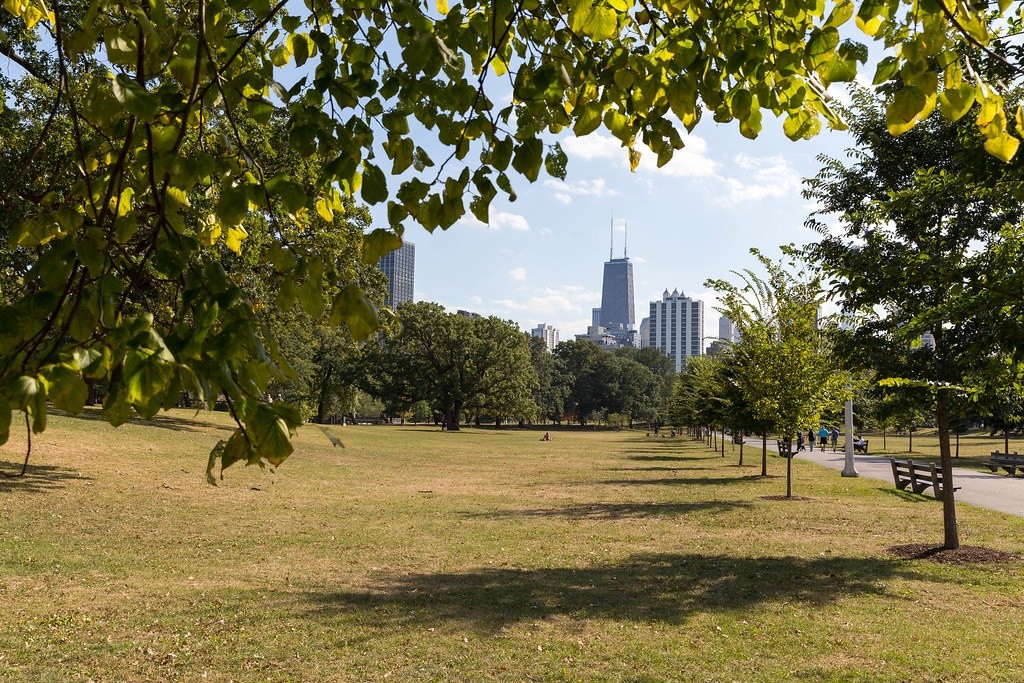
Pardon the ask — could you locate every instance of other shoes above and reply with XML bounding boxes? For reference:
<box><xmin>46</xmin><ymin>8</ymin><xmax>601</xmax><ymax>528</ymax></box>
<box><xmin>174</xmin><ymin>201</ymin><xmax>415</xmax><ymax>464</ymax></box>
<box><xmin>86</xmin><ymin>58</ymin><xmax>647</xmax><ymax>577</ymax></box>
<box><xmin>821</xmin><ymin>448</ymin><xmax>825</xmax><ymax>452</ymax></box>
<box><xmin>833</xmin><ymin>449</ymin><xmax>835</xmax><ymax>452</ymax></box>
<box><xmin>810</xmin><ymin>449</ymin><xmax>812</xmax><ymax>452</ymax></box>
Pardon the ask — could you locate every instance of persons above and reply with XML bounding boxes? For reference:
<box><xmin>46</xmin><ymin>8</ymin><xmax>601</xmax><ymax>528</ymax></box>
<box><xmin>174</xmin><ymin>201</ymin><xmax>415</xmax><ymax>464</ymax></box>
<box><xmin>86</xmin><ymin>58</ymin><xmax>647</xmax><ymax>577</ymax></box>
<box><xmin>830</xmin><ymin>426</ymin><xmax>839</xmax><ymax>452</ymax></box>
<box><xmin>796</xmin><ymin>427</ymin><xmax>806</xmax><ymax>452</ymax></box>
<box><xmin>817</xmin><ymin>425</ymin><xmax>830</xmax><ymax>452</ymax></box>
<box><xmin>670</xmin><ymin>430</ymin><xmax>675</xmax><ymax>438</ymax></box>
<box><xmin>653</xmin><ymin>424</ymin><xmax>659</xmax><ymax>438</ymax></box>
<box><xmin>542</xmin><ymin>432</ymin><xmax>551</xmax><ymax>442</ymax></box>
<box><xmin>807</xmin><ymin>428</ymin><xmax>816</xmax><ymax>452</ymax></box>
<box><xmin>841</xmin><ymin>434</ymin><xmax>865</xmax><ymax>452</ymax></box>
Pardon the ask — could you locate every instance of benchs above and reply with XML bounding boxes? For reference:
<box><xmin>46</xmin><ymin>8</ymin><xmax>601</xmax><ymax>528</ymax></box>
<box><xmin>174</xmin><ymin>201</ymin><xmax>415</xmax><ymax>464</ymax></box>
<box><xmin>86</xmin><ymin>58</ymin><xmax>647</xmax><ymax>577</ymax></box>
<box><xmin>982</xmin><ymin>451</ymin><xmax>1024</xmax><ymax>477</ymax></box>
<box><xmin>890</xmin><ymin>456</ymin><xmax>963</xmax><ymax>501</ymax></box>
<box><xmin>733</xmin><ymin>438</ymin><xmax>745</xmax><ymax>445</ymax></box>
<box><xmin>777</xmin><ymin>440</ymin><xmax>798</xmax><ymax>458</ymax></box>
<box><xmin>850</xmin><ymin>439</ymin><xmax>870</xmax><ymax>454</ymax></box>
